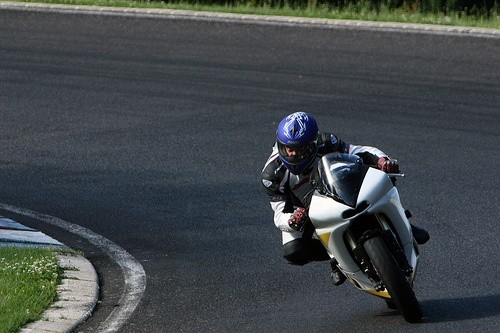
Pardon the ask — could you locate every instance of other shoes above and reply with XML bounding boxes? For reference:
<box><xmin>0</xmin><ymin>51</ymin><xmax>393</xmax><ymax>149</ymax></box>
<box><xmin>410</xmin><ymin>222</ymin><xmax>430</xmax><ymax>245</ymax></box>
<box><xmin>329</xmin><ymin>258</ymin><xmax>346</xmax><ymax>285</ymax></box>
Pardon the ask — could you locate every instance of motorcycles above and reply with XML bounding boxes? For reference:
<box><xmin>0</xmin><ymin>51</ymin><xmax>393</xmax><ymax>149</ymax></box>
<box><xmin>288</xmin><ymin>153</ymin><xmax>422</xmax><ymax>325</ymax></box>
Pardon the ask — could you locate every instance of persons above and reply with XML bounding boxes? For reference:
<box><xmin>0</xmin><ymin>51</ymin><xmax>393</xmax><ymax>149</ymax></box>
<box><xmin>262</xmin><ymin>112</ymin><xmax>430</xmax><ymax>286</ymax></box>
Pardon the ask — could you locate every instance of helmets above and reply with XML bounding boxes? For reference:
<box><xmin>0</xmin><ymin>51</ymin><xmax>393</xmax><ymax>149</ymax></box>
<box><xmin>274</xmin><ymin>110</ymin><xmax>322</xmax><ymax>176</ymax></box>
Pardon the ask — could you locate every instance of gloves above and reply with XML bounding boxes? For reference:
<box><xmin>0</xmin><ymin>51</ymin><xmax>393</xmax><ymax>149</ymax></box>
<box><xmin>378</xmin><ymin>155</ymin><xmax>400</xmax><ymax>174</ymax></box>
<box><xmin>287</xmin><ymin>206</ymin><xmax>305</xmax><ymax>230</ymax></box>
